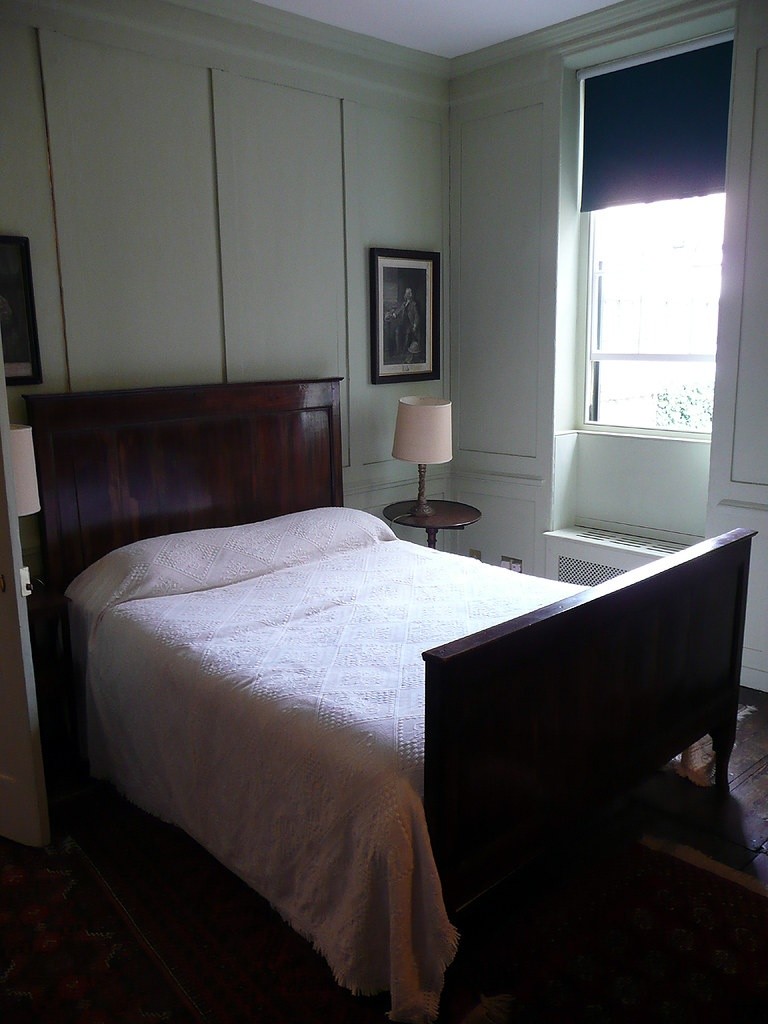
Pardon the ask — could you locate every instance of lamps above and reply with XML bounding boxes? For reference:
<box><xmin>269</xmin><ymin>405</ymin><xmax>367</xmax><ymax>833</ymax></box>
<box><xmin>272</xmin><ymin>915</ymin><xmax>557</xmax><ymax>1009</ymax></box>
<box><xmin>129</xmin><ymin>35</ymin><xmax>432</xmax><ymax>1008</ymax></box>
<box><xmin>392</xmin><ymin>396</ymin><xmax>453</xmax><ymax>517</ymax></box>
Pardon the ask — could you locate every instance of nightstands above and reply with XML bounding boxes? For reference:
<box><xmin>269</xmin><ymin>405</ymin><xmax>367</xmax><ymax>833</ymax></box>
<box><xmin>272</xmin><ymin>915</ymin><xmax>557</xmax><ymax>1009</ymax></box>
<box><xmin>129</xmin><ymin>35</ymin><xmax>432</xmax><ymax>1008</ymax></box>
<box><xmin>383</xmin><ymin>500</ymin><xmax>481</xmax><ymax>550</ymax></box>
<box><xmin>27</xmin><ymin>576</ymin><xmax>89</xmax><ymax>764</ymax></box>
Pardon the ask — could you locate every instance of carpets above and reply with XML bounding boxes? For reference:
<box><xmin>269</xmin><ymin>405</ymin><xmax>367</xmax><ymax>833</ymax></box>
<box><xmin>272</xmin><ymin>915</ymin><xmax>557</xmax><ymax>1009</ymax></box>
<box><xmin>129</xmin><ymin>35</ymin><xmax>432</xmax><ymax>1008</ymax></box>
<box><xmin>0</xmin><ymin>775</ymin><xmax>768</xmax><ymax>1024</ymax></box>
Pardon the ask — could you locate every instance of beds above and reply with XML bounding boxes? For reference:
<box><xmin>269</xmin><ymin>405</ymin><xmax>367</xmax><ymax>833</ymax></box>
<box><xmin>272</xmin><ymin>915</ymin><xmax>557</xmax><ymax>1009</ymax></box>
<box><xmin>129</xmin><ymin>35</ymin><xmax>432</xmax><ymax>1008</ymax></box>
<box><xmin>19</xmin><ymin>376</ymin><xmax>758</xmax><ymax>1024</ymax></box>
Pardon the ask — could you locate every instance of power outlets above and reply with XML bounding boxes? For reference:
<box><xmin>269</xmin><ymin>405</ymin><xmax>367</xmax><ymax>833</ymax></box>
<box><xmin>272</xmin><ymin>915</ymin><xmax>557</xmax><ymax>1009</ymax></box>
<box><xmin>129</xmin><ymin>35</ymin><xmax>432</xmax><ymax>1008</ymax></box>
<box><xmin>469</xmin><ymin>549</ymin><xmax>481</xmax><ymax>561</ymax></box>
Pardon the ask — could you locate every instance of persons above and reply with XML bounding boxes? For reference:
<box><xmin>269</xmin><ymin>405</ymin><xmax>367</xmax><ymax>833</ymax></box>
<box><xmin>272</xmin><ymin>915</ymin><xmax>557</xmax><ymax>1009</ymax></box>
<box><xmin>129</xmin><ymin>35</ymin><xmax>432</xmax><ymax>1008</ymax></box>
<box><xmin>390</xmin><ymin>288</ymin><xmax>420</xmax><ymax>362</ymax></box>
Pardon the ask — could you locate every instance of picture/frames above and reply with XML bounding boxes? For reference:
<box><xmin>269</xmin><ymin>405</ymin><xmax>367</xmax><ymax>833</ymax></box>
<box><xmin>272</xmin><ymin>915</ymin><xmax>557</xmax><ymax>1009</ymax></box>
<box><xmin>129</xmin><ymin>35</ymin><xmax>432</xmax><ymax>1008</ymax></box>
<box><xmin>369</xmin><ymin>247</ymin><xmax>442</xmax><ymax>385</ymax></box>
<box><xmin>0</xmin><ymin>235</ymin><xmax>43</xmax><ymax>387</ymax></box>
